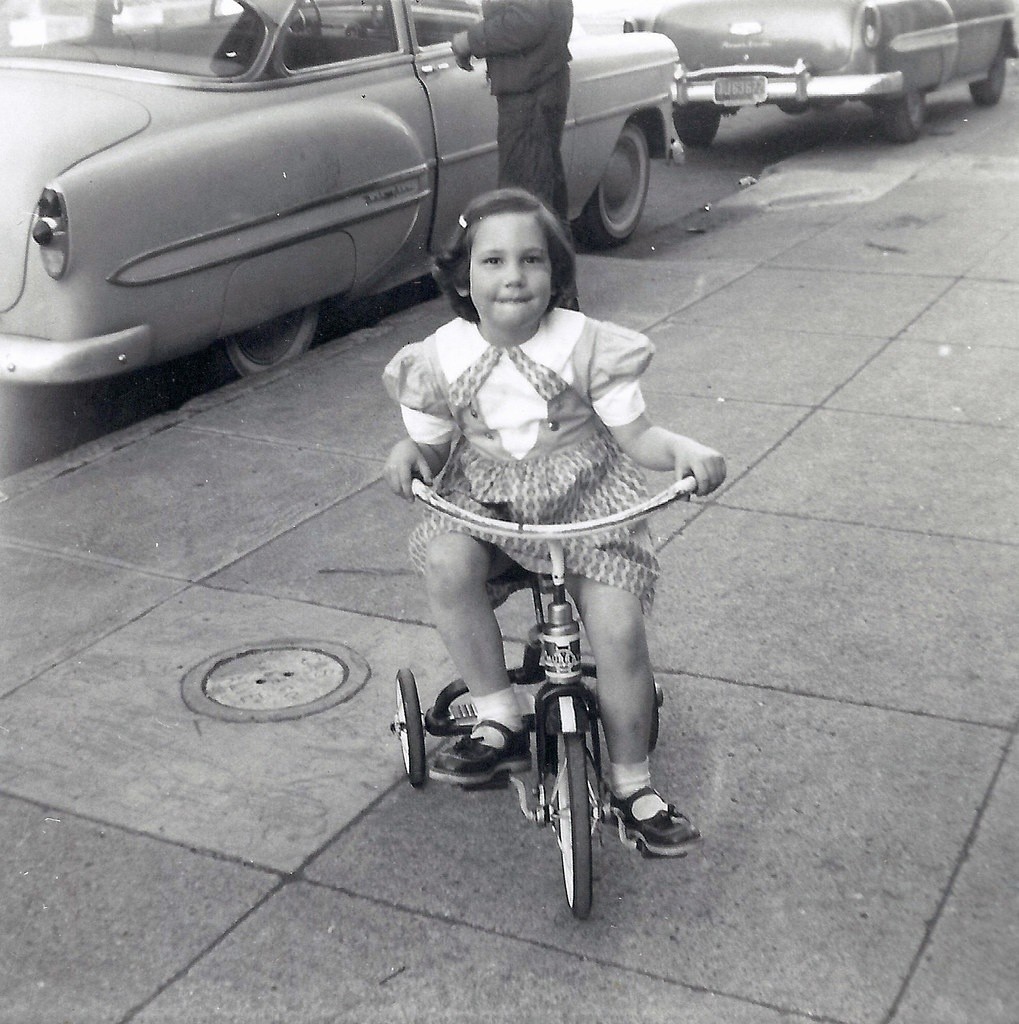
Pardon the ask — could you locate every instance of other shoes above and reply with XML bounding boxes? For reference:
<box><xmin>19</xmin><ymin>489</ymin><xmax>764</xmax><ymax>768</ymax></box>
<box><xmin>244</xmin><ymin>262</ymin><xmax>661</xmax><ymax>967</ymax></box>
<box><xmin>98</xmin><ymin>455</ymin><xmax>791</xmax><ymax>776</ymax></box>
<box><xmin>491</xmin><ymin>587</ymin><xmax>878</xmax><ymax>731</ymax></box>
<box><xmin>428</xmin><ymin>715</ymin><xmax>532</xmax><ymax>784</ymax></box>
<box><xmin>608</xmin><ymin>785</ymin><xmax>704</xmax><ymax>855</ymax></box>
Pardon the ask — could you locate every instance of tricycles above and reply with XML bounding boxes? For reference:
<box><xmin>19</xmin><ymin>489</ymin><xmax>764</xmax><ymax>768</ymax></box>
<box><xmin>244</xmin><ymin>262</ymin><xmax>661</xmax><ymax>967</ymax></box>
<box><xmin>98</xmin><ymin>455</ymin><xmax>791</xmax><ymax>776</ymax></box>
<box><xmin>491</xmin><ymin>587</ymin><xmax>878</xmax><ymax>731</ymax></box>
<box><xmin>389</xmin><ymin>473</ymin><xmax>701</xmax><ymax>919</ymax></box>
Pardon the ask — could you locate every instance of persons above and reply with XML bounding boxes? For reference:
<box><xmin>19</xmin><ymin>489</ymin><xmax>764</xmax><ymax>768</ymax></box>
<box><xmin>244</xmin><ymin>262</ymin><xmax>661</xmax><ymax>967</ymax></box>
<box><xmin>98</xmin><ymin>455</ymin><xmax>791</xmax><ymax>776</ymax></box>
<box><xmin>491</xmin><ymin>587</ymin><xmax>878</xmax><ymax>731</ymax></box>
<box><xmin>447</xmin><ymin>0</ymin><xmax>581</xmax><ymax>312</ymax></box>
<box><xmin>377</xmin><ymin>187</ymin><xmax>729</xmax><ymax>856</ymax></box>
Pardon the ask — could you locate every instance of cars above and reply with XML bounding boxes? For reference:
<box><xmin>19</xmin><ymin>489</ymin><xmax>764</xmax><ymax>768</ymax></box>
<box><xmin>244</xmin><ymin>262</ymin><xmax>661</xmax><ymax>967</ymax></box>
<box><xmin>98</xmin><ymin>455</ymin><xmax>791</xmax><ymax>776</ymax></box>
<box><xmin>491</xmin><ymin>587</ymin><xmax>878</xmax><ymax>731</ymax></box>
<box><xmin>623</xmin><ymin>0</ymin><xmax>1019</xmax><ymax>145</ymax></box>
<box><xmin>0</xmin><ymin>0</ymin><xmax>688</xmax><ymax>389</ymax></box>
